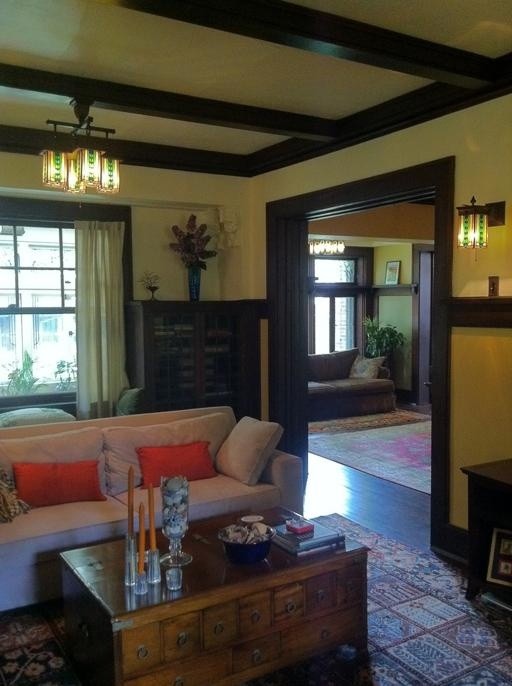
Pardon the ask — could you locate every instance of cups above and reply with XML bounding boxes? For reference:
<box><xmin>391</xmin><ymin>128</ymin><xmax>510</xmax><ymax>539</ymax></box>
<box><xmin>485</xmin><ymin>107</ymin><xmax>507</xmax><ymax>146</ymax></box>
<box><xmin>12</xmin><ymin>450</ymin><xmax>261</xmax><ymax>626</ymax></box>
<box><xmin>165</xmin><ymin>569</ymin><xmax>184</xmax><ymax>591</ymax></box>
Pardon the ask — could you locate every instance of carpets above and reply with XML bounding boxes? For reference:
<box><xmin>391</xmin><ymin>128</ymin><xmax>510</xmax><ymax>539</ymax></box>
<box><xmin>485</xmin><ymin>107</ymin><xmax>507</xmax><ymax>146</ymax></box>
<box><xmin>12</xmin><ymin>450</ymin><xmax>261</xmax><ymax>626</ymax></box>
<box><xmin>308</xmin><ymin>410</ymin><xmax>431</xmax><ymax>499</ymax></box>
<box><xmin>0</xmin><ymin>513</ymin><xmax>512</xmax><ymax>686</ymax></box>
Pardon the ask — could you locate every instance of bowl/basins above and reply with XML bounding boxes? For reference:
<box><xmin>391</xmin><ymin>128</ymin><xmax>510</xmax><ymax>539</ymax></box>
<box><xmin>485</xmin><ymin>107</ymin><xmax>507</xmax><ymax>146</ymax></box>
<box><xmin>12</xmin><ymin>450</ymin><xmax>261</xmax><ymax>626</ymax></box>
<box><xmin>219</xmin><ymin>526</ymin><xmax>277</xmax><ymax>563</ymax></box>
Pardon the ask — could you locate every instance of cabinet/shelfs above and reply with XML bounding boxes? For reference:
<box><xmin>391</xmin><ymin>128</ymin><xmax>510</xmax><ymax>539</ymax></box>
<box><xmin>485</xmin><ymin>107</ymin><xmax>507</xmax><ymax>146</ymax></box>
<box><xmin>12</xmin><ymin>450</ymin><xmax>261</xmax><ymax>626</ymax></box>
<box><xmin>126</xmin><ymin>299</ymin><xmax>266</xmax><ymax>418</ymax></box>
<box><xmin>59</xmin><ymin>502</ymin><xmax>370</xmax><ymax>686</ymax></box>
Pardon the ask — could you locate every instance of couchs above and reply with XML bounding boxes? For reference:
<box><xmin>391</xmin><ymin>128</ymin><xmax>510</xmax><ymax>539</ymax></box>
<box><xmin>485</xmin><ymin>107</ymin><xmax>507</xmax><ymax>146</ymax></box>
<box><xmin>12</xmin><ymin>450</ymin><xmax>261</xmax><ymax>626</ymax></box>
<box><xmin>307</xmin><ymin>349</ymin><xmax>397</xmax><ymax>422</ymax></box>
<box><xmin>0</xmin><ymin>405</ymin><xmax>303</xmax><ymax>611</ymax></box>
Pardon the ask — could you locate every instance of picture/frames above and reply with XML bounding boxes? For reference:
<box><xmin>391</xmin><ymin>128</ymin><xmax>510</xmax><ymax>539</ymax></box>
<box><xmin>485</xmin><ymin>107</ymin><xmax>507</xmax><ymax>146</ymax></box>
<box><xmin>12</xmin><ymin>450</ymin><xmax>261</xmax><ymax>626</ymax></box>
<box><xmin>483</xmin><ymin>527</ymin><xmax>512</xmax><ymax>588</ymax></box>
<box><xmin>385</xmin><ymin>261</ymin><xmax>401</xmax><ymax>285</ymax></box>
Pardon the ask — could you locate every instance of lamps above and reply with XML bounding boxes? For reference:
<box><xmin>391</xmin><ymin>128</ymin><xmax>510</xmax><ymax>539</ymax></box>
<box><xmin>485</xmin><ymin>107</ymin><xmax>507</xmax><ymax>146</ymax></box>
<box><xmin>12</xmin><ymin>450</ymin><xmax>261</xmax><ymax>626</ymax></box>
<box><xmin>38</xmin><ymin>96</ymin><xmax>124</xmax><ymax>195</ymax></box>
<box><xmin>456</xmin><ymin>194</ymin><xmax>505</xmax><ymax>249</ymax></box>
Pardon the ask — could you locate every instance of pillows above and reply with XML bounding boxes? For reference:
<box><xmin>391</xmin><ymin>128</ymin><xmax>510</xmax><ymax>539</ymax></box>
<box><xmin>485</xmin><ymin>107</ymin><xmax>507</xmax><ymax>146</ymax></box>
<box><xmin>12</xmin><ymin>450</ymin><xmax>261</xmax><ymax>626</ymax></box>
<box><xmin>0</xmin><ymin>412</ymin><xmax>283</xmax><ymax>508</ymax></box>
<box><xmin>348</xmin><ymin>353</ymin><xmax>387</xmax><ymax>380</ymax></box>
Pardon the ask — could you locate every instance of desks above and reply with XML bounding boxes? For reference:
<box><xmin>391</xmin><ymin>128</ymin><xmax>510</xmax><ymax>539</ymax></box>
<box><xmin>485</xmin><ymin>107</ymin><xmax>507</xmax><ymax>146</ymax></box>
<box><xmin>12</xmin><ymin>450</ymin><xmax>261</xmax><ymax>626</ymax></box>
<box><xmin>459</xmin><ymin>456</ymin><xmax>512</xmax><ymax>613</ymax></box>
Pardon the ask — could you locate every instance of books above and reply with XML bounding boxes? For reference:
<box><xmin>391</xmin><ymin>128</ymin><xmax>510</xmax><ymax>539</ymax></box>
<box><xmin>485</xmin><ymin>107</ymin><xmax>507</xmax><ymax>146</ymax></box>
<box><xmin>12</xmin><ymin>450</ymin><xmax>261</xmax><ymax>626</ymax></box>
<box><xmin>269</xmin><ymin>519</ymin><xmax>346</xmax><ymax>559</ymax></box>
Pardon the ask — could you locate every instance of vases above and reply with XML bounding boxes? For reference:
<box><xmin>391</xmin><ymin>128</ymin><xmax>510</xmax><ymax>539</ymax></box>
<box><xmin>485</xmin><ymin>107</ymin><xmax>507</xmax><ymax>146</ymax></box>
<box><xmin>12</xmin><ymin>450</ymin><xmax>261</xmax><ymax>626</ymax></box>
<box><xmin>187</xmin><ymin>265</ymin><xmax>201</xmax><ymax>301</ymax></box>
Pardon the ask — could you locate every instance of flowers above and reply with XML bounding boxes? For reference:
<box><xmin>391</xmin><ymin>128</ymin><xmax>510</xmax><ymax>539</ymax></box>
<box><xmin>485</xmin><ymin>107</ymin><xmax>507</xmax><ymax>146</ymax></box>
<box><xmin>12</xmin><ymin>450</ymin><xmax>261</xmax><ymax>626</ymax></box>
<box><xmin>166</xmin><ymin>213</ymin><xmax>219</xmax><ymax>271</ymax></box>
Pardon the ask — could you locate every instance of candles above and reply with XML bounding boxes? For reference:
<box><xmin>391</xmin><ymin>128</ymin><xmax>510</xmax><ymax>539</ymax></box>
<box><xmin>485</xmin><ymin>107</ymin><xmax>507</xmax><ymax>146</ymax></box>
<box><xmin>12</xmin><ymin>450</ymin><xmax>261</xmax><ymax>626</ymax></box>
<box><xmin>125</xmin><ymin>464</ymin><xmax>156</xmax><ymax>576</ymax></box>
